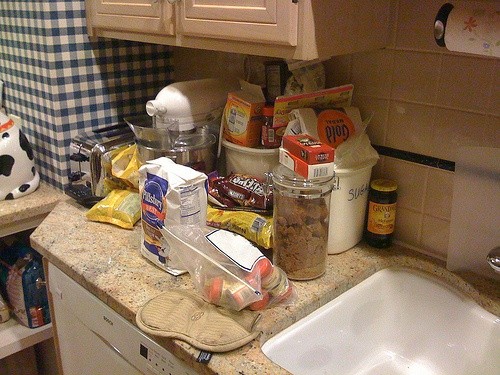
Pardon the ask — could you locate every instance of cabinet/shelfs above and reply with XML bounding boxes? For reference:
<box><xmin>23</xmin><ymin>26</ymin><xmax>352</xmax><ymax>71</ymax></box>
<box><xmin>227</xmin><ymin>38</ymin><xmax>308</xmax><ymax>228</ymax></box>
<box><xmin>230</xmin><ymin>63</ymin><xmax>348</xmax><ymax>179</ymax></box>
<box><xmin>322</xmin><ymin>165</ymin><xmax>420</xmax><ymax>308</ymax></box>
<box><xmin>84</xmin><ymin>0</ymin><xmax>390</xmax><ymax>61</ymax></box>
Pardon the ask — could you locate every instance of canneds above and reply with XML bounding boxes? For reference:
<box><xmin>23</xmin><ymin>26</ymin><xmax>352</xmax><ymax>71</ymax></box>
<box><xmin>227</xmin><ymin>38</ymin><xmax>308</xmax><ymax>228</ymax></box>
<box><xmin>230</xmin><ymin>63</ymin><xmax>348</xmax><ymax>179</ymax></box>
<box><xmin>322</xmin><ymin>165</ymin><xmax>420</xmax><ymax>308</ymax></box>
<box><xmin>206</xmin><ymin>260</ymin><xmax>292</xmax><ymax>312</ymax></box>
<box><xmin>265</xmin><ymin>164</ymin><xmax>340</xmax><ymax>281</ymax></box>
<box><xmin>365</xmin><ymin>179</ymin><xmax>398</xmax><ymax>248</ymax></box>
<box><xmin>260</xmin><ymin>106</ymin><xmax>286</xmax><ymax>148</ymax></box>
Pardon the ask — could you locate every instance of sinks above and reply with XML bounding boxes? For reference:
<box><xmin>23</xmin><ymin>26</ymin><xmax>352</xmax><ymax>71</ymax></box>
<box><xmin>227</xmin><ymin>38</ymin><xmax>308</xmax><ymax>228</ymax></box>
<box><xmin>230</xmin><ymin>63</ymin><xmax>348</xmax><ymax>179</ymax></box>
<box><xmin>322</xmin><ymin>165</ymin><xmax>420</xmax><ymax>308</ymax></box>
<box><xmin>260</xmin><ymin>264</ymin><xmax>500</xmax><ymax>375</ymax></box>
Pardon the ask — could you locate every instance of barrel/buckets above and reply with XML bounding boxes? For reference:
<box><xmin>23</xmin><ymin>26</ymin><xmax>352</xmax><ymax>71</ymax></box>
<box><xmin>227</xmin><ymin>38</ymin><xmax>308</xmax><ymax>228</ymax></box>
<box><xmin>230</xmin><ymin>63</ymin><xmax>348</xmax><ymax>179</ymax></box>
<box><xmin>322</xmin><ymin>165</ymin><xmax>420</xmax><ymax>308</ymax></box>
<box><xmin>223</xmin><ymin>138</ymin><xmax>281</xmax><ymax>183</ymax></box>
<box><xmin>325</xmin><ymin>160</ymin><xmax>380</xmax><ymax>254</ymax></box>
<box><xmin>134</xmin><ymin>130</ymin><xmax>218</xmax><ymax>176</ymax></box>
